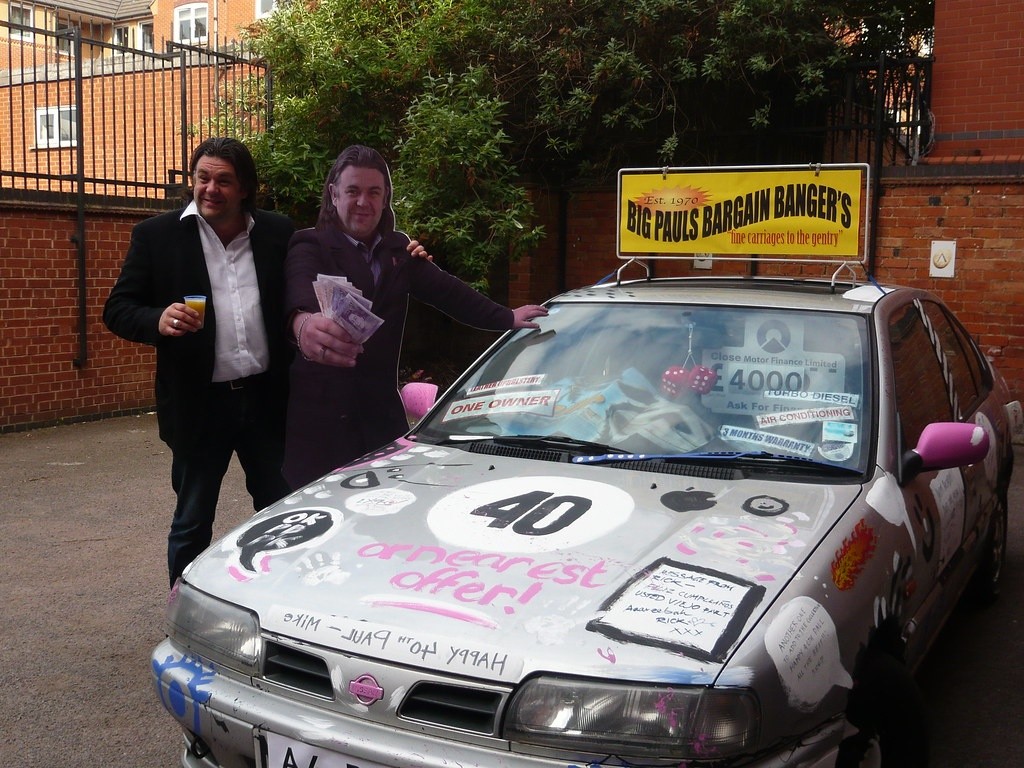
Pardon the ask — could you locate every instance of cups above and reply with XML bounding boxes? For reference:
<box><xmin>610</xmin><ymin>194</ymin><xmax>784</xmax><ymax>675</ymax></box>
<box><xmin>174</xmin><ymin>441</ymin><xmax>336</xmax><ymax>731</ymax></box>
<box><xmin>183</xmin><ymin>296</ymin><xmax>205</xmax><ymax>328</ymax></box>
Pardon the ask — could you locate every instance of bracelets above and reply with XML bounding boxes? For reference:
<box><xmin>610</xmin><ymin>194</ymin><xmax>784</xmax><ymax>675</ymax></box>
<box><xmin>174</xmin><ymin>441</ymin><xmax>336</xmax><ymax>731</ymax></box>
<box><xmin>297</xmin><ymin>312</ymin><xmax>320</xmax><ymax>361</ymax></box>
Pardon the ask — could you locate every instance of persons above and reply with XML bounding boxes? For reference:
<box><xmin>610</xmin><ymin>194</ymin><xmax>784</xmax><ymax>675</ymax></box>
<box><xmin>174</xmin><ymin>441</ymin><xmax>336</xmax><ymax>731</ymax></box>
<box><xmin>102</xmin><ymin>137</ymin><xmax>433</xmax><ymax>591</ymax></box>
<box><xmin>284</xmin><ymin>144</ymin><xmax>549</xmax><ymax>496</ymax></box>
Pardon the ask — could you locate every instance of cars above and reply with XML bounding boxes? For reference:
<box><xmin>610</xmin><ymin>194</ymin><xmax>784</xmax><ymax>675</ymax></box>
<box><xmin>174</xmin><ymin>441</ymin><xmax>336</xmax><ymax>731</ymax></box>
<box><xmin>153</xmin><ymin>276</ymin><xmax>1022</xmax><ymax>768</ymax></box>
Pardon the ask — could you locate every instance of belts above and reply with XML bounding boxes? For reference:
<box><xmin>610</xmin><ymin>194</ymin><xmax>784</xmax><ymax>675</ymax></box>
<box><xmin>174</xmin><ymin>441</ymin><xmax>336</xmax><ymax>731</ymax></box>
<box><xmin>208</xmin><ymin>369</ymin><xmax>272</xmax><ymax>392</ymax></box>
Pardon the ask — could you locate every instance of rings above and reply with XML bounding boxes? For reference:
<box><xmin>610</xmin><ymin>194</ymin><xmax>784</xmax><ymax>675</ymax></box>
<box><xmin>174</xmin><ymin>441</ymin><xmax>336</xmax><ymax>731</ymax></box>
<box><xmin>319</xmin><ymin>347</ymin><xmax>327</xmax><ymax>360</ymax></box>
<box><xmin>173</xmin><ymin>319</ymin><xmax>180</xmax><ymax>327</ymax></box>
